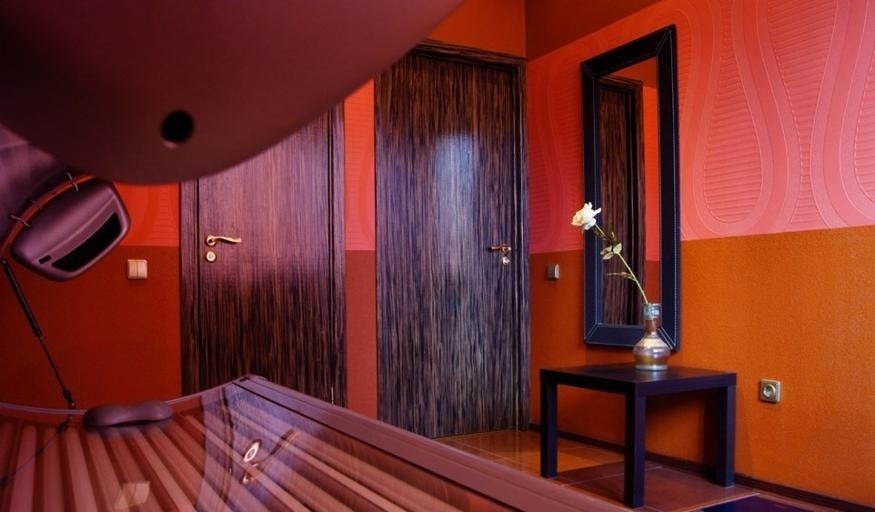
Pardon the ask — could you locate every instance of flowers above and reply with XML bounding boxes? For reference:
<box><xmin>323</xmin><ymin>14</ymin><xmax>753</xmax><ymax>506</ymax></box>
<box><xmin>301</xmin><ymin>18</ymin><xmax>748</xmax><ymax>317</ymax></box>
<box><xmin>571</xmin><ymin>203</ymin><xmax>658</xmax><ymax>327</ymax></box>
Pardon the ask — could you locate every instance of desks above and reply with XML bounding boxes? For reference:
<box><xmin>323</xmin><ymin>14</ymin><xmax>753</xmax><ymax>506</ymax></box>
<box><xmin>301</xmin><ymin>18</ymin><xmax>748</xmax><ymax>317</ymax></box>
<box><xmin>539</xmin><ymin>364</ymin><xmax>737</xmax><ymax>507</ymax></box>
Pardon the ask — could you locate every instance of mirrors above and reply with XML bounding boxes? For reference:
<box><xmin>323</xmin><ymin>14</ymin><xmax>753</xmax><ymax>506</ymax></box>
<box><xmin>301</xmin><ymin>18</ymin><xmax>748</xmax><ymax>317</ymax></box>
<box><xmin>579</xmin><ymin>25</ymin><xmax>680</xmax><ymax>351</ymax></box>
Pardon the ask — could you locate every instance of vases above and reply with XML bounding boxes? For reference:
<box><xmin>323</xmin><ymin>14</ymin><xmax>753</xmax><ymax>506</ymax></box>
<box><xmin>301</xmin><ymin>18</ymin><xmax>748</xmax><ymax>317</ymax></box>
<box><xmin>634</xmin><ymin>306</ymin><xmax>672</xmax><ymax>369</ymax></box>
